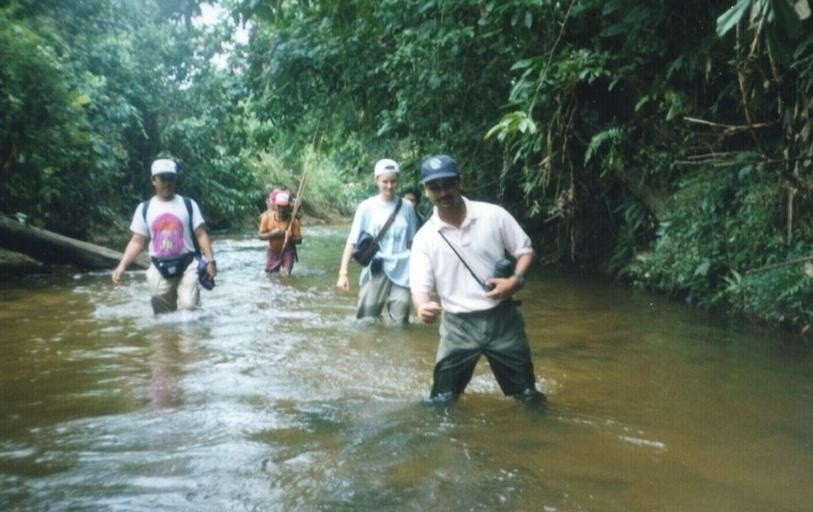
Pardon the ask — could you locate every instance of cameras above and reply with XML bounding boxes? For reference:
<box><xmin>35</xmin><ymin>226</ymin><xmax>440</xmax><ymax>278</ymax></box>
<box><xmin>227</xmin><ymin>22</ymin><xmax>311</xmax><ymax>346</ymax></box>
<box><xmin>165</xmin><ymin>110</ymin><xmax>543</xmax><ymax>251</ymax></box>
<box><xmin>482</xmin><ymin>258</ymin><xmax>513</xmax><ymax>292</ymax></box>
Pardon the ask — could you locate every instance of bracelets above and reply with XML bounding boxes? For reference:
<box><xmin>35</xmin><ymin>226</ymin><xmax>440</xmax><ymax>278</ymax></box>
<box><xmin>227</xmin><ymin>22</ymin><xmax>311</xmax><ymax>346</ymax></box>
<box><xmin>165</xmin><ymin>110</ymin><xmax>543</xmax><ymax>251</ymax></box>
<box><xmin>205</xmin><ymin>260</ymin><xmax>215</xmax><ymax>266</ymax></box>
<box><xmin>338</xmin><ymin>270</ymin><xmax>347</xmax><ymax>274</ymax></box>
<box><xmin>509</xmin><ymin>274</ymin><xmax>527</xmax><ymax>286</ymax></box>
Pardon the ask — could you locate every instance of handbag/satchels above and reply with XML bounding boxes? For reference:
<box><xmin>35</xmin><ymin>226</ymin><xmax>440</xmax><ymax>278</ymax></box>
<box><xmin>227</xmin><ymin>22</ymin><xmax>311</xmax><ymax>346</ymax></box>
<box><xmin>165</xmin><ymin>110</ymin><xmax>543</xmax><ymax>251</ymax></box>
<box><xmin>152</xmin><ymin>253</ymin><xmax>194</xmax><ymax>278</ymax></box>
<box><xmin>353</xmin><ymin>231</ymin><xmax>381</xmax><ymax>267</ymax></box>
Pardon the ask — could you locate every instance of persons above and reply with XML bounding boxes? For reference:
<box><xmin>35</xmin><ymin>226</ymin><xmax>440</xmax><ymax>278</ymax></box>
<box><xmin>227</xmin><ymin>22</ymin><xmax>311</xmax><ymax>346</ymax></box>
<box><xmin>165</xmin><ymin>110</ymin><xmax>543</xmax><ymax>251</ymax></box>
<box><xmin>108</xmin><ymin>157</ymin><xmax>217</xmax><ymax>314</ymax></box>
<box><xmin>335</xmin><ymin>158</ymin><xmax>418</xmax><ymax>327</ymax></box>
<box><xmin>258</xmin><ymin>189</ymin><xmax>302</xmax><ymax>277</ymax></box>
<box><xmin>408</xmin><ymin>155</ymin><xmax>549</xmax><ymax>404</ymax></box>
<box><xmin>399</xmin><ymin>184</ymin><xmax>427</xmax><ymax>226</ymax></box>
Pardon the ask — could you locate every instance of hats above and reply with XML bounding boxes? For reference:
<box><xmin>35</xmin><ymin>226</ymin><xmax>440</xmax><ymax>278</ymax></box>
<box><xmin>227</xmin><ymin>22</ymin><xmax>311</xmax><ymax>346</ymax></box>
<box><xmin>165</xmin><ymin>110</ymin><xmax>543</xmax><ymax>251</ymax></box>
<box><xmin>374</xmin><ymin>158</ymin><xmax>401</xmax><ymax>178</ymax></box>
<box><xmin>151</xmin><ymin>159</ymin><xmax>177</xmax><ymax>176</ymax></box>
<box><xmin>275</xmin><ymin>193</ymin><xmax>290</xmax><ymax>206</ymax></box>
<box><xmin>419</xmin><ymin>154</ymin><xmax>459</xmax><ymax>185</ymax></box>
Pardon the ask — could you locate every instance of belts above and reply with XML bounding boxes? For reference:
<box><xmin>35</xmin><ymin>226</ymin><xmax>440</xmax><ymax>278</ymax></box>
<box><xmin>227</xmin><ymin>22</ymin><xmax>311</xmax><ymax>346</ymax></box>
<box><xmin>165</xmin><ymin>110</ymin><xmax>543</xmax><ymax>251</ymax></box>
<box><xmin>444</xmin><ymin>296</ymin><xmax>522</xmax><ymax>317</ymax></box>
